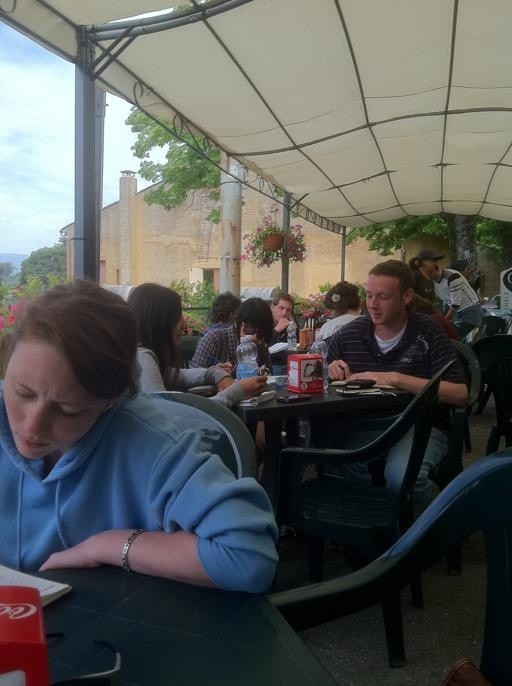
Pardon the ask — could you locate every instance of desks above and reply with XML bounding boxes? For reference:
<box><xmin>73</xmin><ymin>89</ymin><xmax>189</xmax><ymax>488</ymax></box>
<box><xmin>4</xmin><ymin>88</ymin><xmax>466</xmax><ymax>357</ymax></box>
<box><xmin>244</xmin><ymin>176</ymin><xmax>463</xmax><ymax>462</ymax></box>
<box><xmin>159</xmin><ymin>314</ymin><xmax>511</xmax><ymax>461</ymax></box>
<box><xmin>7</xmin><ymin>564</ymin><xmax>346</xmax><ymax>686</ymax></box>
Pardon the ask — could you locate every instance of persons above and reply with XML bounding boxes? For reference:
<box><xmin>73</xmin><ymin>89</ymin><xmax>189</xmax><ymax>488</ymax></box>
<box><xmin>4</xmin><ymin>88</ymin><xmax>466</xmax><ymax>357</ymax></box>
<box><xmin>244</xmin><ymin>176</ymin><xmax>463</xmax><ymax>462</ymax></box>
<box><xmin>326</xmin><ymin>259</ymin><xmax>468</xmax><ymax>517</ymax></box>
<box><xmin>321</xmin><ymin>281</ymin><xmax>365</xmax><ymax>339</ymax></box>
<box><xmin>408</xmin><ymin>248</ymin><xmax>484</xmax><ymax>341</ymax></box>
<box><xmin>1</xmin><ymin>281</ymin><xmax>280</xmax><ymax>593</ymax></box>
<box><xmin>127</xmin><ymin>282</ymin><xmax>268</xmax><ymax>408</ymax></box>
<box><xmin>270</xmin><ymin>291</ymin><xmax>299</xmax><ymax>364</ymax></box>
<box><xmin>189</xmin><ymin>291</ymin><xmax>273</xmax><ymax>377</ymax></box>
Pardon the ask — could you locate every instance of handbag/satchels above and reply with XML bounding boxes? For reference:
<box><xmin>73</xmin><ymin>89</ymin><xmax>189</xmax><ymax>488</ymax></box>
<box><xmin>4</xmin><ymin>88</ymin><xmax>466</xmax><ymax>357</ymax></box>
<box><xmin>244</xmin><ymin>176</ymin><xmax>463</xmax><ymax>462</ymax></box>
<box><xmin>188</xmin><ymin>386</ymin><xmax>217</xmax><ymax>396</ymax></box>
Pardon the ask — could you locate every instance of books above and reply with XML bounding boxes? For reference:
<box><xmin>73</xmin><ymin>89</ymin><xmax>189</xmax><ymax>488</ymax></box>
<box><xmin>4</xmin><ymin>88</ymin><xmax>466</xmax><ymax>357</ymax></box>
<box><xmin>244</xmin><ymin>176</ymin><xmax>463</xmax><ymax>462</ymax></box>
<box><xmin>0</xmin><ymin>565</ymin><xmax>74</xmax><ymax>608</ymax></box>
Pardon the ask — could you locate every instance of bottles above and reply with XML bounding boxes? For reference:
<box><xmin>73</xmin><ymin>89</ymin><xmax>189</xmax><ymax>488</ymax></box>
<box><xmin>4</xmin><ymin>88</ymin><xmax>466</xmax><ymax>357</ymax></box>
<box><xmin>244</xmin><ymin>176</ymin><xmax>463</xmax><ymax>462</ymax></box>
<box><xmin>298</xmin><ymin>328</ymin><xmax>313</xmax><ymax>349</ymax></box>
<box><xmin>310</xmin><ymin>334</ymin><xmax>330</xmax><ymax>391</ymax></box>
<box><xmin>286</xmin><ymin>321</ymin><xmax>296</xmax><ymax>348</ymax></box>
<box><xmin>244</xmin><ymin>335</ymin><xmax>258</xmax><ymax>377</ymax></box>
<box><xmin>236</xmin><ymin>337</ymin><xmax>256</xmax><ymax>403</ymax></box>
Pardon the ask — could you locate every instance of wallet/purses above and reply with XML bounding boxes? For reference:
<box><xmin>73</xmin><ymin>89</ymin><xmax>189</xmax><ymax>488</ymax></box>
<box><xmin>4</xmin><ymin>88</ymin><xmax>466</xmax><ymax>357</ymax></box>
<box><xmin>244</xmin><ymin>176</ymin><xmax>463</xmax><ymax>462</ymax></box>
<box><xmin>344</xmin><ymin>379</ymin><xmax>376</xmax><ymax>389</ymax></box>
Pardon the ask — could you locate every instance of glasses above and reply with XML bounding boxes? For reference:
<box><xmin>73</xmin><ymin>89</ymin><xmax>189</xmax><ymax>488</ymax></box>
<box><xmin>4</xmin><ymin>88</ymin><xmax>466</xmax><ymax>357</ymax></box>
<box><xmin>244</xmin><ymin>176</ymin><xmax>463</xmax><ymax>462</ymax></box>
<box><xmin>47</xmin><ymin>632</ymin><xmax>121</xmax><ymax>685</ymax></box>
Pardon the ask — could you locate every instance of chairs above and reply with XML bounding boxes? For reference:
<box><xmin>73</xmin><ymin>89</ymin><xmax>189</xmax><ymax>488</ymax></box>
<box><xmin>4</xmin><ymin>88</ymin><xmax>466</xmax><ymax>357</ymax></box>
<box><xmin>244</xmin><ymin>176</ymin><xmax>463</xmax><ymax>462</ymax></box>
<box><xmin>122</xmin><ymin>390</ymin><xmax>257</xmax><ymax>487</ymax></box>
<box><xmin>276</xmin><ymin>356</ymin><xmax>458</xmax><ymax>667</ymax></box>
<box><xmin>268</xmin><ymin>447</ymin><xmax>511</xmax><ymax>685</ymax></box>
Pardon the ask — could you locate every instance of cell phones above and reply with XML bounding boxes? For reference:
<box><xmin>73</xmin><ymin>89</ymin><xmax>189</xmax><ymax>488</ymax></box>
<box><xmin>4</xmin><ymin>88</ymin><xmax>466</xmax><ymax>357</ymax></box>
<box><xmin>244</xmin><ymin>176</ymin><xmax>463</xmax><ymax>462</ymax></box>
<box><xmin>276</xmin><ymin>394</ymin><xmax>312</xmax><ymax>404</ymax></box>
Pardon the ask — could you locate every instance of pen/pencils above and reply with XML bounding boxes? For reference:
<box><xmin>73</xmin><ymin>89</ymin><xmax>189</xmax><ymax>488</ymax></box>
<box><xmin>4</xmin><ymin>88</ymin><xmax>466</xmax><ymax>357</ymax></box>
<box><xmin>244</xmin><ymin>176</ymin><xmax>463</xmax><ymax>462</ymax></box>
<box><xmin>338</xmin><ymin>365</ymin><xmax>345</xmax><ymax>371</ymax></box>
<box><xmin>258</xmin><ymin>371</ymin><xmax>260</xmax><ymax>376</ymax></box>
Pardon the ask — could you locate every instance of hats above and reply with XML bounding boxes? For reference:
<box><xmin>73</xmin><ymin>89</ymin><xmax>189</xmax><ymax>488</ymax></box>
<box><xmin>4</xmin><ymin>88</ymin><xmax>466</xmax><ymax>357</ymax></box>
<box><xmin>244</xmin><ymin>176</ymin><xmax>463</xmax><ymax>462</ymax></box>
<box><xmin>417</xmin><ymin>249</ymin><xmax>444</xmax><ymax>260</ymax></box>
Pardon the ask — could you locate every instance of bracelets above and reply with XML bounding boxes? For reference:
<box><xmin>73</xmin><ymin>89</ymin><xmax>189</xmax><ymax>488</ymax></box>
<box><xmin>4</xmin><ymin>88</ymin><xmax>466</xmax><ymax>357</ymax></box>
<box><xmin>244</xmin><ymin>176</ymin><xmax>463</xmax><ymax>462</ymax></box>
<box><xmin>122</xmin><ymin>529</ymin><xmax>149</xmax><ymax>571</ymax></box>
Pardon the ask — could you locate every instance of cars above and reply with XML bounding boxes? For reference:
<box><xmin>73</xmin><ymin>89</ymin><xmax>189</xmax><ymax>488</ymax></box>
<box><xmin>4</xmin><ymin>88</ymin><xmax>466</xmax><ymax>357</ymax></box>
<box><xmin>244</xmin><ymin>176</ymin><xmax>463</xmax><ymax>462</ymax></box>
<box><xmin>480</xmin><ymin>295</ymin><xmax>500</xmax><ymax>315</ymax></box>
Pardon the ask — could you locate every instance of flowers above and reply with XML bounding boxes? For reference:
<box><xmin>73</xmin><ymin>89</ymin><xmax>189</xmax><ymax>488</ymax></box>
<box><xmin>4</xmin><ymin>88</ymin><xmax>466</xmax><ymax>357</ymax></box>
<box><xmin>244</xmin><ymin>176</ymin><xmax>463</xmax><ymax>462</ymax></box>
<box><xmin>243</xmin><ymin>205</ymin><xmax>307</xmax><ymax>268</ymax></box>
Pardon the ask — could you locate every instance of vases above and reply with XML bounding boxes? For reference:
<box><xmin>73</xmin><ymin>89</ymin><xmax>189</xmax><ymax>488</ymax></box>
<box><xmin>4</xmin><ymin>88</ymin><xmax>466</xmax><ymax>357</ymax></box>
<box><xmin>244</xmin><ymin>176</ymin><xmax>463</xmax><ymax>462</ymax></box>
<box><xmin>261</xmin><ymin>230</ymin><xmax>285</xmax><ymax>252</ymax></box>
